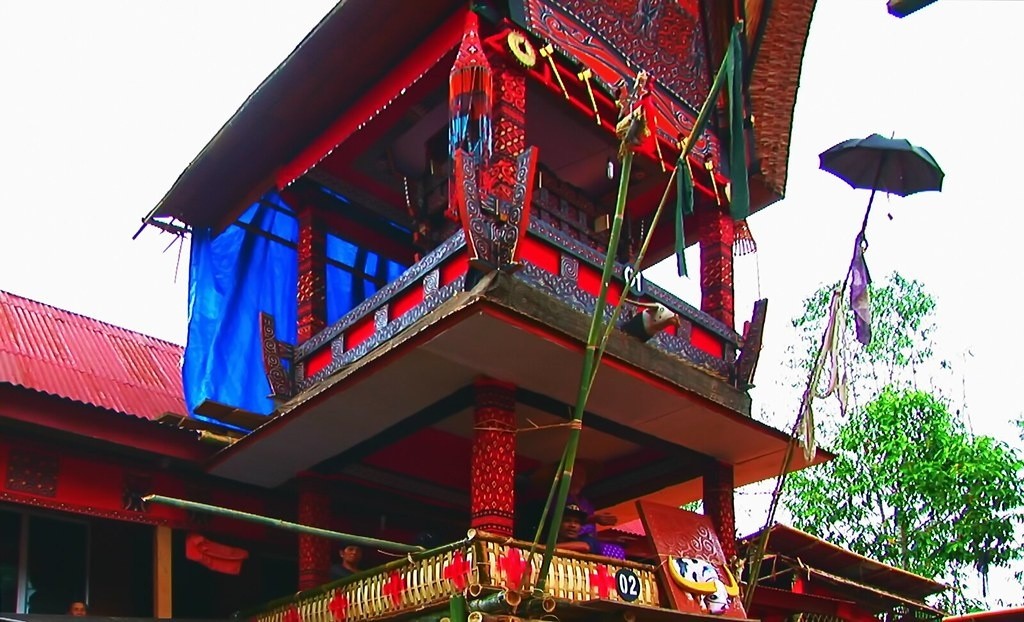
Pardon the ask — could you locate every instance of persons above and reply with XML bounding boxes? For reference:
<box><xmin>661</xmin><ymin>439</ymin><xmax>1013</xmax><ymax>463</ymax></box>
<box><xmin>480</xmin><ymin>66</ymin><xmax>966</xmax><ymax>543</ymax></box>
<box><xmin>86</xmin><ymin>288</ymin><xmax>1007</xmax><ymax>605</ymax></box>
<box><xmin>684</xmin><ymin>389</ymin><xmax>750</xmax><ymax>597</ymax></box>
<box><xmin>68</xmin><ymin>601</ymin><xmax>90</xmax><ymax>618</ymax></box>
<box><xmin>328</xmin><ymin>541</ymin><xmax>373</xmax><ymax>580</ymax></box>
<box><xmin>554</xmin><ymin>504</ymin><xmax>602</xmax><ymax>555</ymax></box>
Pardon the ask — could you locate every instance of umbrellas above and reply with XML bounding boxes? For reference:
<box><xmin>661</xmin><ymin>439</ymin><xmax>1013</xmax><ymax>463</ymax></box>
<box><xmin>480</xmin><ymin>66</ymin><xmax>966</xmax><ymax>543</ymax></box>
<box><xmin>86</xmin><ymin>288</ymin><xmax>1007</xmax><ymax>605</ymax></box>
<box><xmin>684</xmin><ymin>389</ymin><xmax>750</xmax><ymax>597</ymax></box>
<box><xmin>819</xmin><ymin>133</ymin><xmax>945</xmax><ymax>230</ymax></box>
